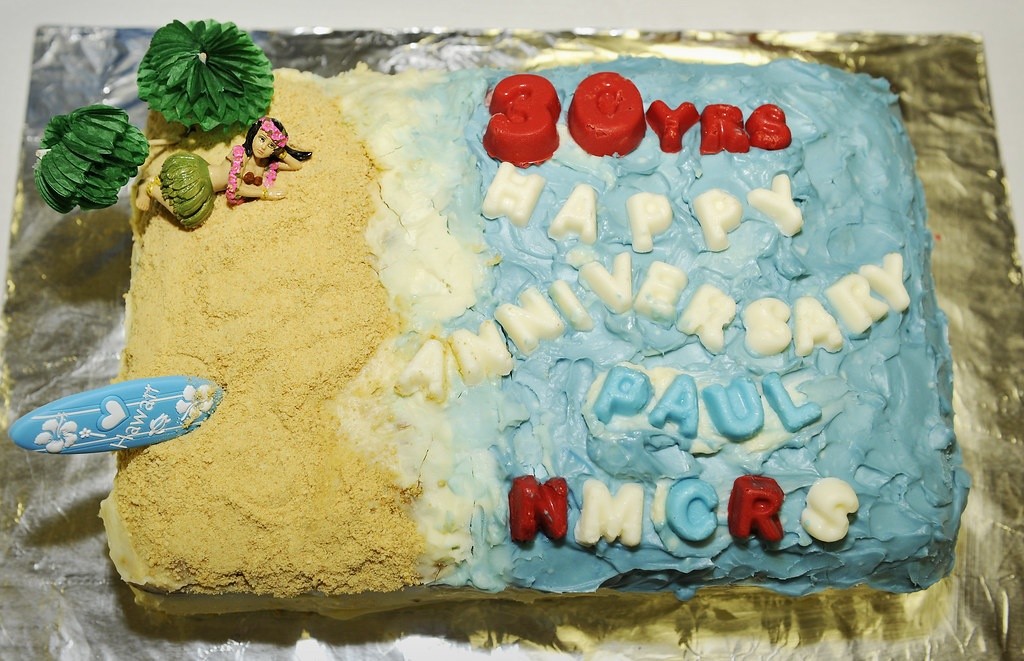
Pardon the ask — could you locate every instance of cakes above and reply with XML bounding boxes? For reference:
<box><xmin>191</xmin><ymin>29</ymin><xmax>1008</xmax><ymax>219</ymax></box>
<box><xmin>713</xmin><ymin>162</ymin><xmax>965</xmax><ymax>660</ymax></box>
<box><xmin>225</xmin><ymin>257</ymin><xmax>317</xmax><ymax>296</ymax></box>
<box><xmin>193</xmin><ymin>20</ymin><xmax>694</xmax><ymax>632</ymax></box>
<box><xmin>9</xmin><ymin>20</ymin><xmax>971</xmax><ymax>623</ymax></box>
